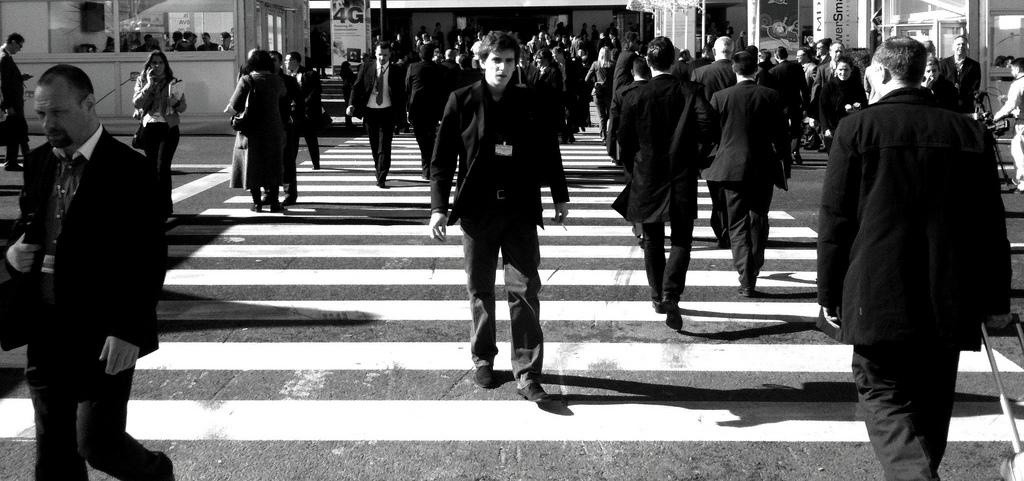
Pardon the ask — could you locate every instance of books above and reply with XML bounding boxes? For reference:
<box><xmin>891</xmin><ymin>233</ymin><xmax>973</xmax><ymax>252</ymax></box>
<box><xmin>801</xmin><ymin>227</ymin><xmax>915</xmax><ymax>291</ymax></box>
<box><xmin>166</xmin><ymin>80</ymin><xmax>185</xmax><ymax>116</ymax></box>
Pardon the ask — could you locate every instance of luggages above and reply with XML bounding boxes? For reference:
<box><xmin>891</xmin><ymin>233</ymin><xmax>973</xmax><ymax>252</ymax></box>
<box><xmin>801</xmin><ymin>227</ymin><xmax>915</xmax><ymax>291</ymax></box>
<box><xmin>981</xmin><ymin>312</ymin><xmax>1024</xmax><ymax>481</ymax></box>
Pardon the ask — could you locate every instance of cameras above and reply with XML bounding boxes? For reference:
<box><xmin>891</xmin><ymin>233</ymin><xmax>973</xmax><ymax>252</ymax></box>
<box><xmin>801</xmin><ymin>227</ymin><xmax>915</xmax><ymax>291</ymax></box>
<box><xmin>974</xmin><ymin>90</ymin><xmax>1010</xmax><ymax>136</ymax></box>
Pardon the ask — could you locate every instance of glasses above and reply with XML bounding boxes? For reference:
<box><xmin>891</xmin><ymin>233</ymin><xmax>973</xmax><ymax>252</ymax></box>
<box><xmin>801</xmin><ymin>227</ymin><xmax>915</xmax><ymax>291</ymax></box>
<box><xmin>14</xmin><ymin>40</ymin><xmax>23</xmax><ymax>49</ymax></box>
<box><xmin>816</xmin><ymin>46</ymin><xmax>824</xmax><ymax>50</ymax></box>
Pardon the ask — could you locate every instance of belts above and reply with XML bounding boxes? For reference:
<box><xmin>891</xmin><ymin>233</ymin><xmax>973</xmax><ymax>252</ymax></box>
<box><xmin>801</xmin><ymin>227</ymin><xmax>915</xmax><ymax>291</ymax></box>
<box><xmin>493</xmin><ymin>188</ymin><xmax>509</xmax><ymax>200</ymax></box>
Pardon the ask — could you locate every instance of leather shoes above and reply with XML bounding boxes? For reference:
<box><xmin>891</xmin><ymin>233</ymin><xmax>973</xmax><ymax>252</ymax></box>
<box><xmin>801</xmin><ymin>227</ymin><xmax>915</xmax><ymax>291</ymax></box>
<box><xmin>473</xmin><ymin>365</ymin><xmax>497</xmax><ymax>390</ymax></box>
<box><xmin>280</xmin><ymin>197</ymin><xmax>296</xmax><ymax>207</ymax></box>
<box><xmin>652</xmin><ymin>298</ymin><xmax>666</xmax><ymax>314</ymax></box>
<box><xmin>794</xmin><ymin>150</ymin><xmax>803</xmax><ymax>166</ymax></box>
<box><xmin>602</xmin><ymin>139</ymin><xmax>607</xmax><ymax>145</ymax></box>
<box><xmin>311</xmin><ymin>165</ymin><xmax>320</xmax><ymax>170</ymax></box>
<box><xmin>270</xmin><ymin>202</ymin><xmax>289</xmax><ymax>213</ymax></box>
<box><xmin>518</xmin><ymin>382</ymin><xmax>552</xmax><ymax>404</ymax></box>
<box><xmin>737</xmin><ymin>285</ymin><xmax>754</xmax><ymax>297</ymax></box>
<box><xmin>377</xmin><ymin>174</ymin><xmax>384</xmax><ymax>187</ymax></box>
<box><xmin>661</xmin><ymin>294</ymin><xmax>684</xmax><ymax>331</ymax></box>
<box><xmin>5</xmin><ymin>163</ymin><xmax>23</xmax><ymax>172</ymax></box>
<box><xmin>250</xmin><ymin>202</ymin><xmax>262</xmax><ymax>212</ymax></box>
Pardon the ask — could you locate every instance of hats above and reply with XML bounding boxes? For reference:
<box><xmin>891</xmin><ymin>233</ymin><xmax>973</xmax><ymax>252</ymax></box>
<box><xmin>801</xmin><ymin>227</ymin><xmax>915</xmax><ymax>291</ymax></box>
<box><xmin>818</xmin><ymin>37</ymin><xmax>832</xmax><ymax>50</ymax></box>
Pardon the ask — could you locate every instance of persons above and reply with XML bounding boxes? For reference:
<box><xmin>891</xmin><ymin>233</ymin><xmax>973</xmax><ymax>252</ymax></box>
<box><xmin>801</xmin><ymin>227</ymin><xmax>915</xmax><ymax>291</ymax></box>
<box><xmin>339</xmin><ymin>61</ymin><xmax>357</xmax><ymax>128</ymax></box>
<box><xmin>171</xmin><ymin>29</ymin><xmax>196</xmax><ymax>50</ymax></box>
<box><xmin>3</xmin><ymin>65</ymin><xmax>175</xmax><ymax>480</ymax></box>
<box><xmin>428</xmin><ymin>30</ymin><xmax>569</xmax><ymax>403</ymax></box>
<box><xmin>231</xmin><ymin>48</ymin><xmax>303</xmax><ymax>214</ymax></box>
<box><xmin>122</xmin><ymin>29</ymin><xmax>162</xmax><ymax>56</ymax></box>
<box><xmin>925</xmin><ymin>54</ymin><xmax>946</xmax><ymax>97</ymax></box>
<box><xmin>597</xmin><ymin>13</ymin><xmax>655</xmax><ymax>48</ymax></box>
<box><xmin>583</xmin><ymin>46</ymin><xmax>615</xmax><ymax>146</ymax></box>
<box><xmin>767</xmin><ymin>46</ymin><xmax>811</xmax><ymax>166</ymax></box>
<box><xmin>390</xmin><ymin>23</ymin><xmax>485</xmax><ymax>72</ymax></box>
<box><xmin>758</xmin><ymin>49</ymin><xmax>776</xmax><ymax>72</ymax></box>
<box><xmin>517</xmin><ymin>21</ymin><xmax>588</xmax><ymax>140</ymax></box>
<box><xmin>816</xmin><ymin>39</ymin><xmax>832</xmax><ymax>66</ymax></box>
<box><xmin>937</xmin><ymin>36</ymin><xmax>982</xmax><ymax>111</ymax></box>
<box><xmin>807</xmin><ymin>59</ymin><xmax>867</xmax><ymax>150</ymax></box>
<box><xmin>795</xmin><ymin>48</ymin><xmax>817</xmax><ymax>84</ymax></box>
<box><xmin>345</xmin><ymin>40</ymin><xmax>405</xmax><ymax>188</ymax></box>
<box><xmin>133</xmin><ymin>49</ymin><xmax>186</xmax><ymax>218</ymax></box>
<box><xmin>994</xmin><ymin>57</ymin><xmax>1024</xmax><ymax>192</ymax></box>
<box><xmin>811</xmin><ymin>43</ymin><xmax>846</xmax><ymax>105</ymax></box>
<box><xmin>197</xmin><ymin>33</ymin><xmax>216</xmax><ymax>50</ymax></box>
<box><xmin>0</xmin><ymin>32</ymin><xmax>35</xmax><ymax>170</ymax></box>
<box><xmin>401</xmin><ymin>41</ymin><xmax>448</xmax><ymax>176</ymax></box>
<box><xmin>260</xmin><ymin>52</ymin><xmax>304</xmax><ymax>206</ymax></box>
<box><xmin>704</xmin><ymin>48</ymin><xmax>791</xmax><ymax>297</ymax></box>
<box><xmin>284</xmin><ymin>52</ymin><xmax>321</xmax><ymax>168</ymax></box>
<box><xmin>689</xmin><ymin>36</ymin><xmax>734</xmax><ymax>248</ymax></box>
<box><xmin>611</xmin><ymin>36</ymin><xmax>717</xmax><ymax>315</ymax></box>
<box><xmin>607</xmin><ymin>57</ymin><xmax>651</xmax><ymax>164</ymax></box>
<box><xmin>676</xmin><ymin>50</ymin><xmax>691</xmax><ymax>80</ymax></box>
<box><xmin>218</xmin><ymin>33</ymin><xmax>236</xmax><ymax>50</ymax></box>
<box><xmin>815</xmin><ymin>36</ymin><xmax>1014</xmax><ymax>480</ymax></box>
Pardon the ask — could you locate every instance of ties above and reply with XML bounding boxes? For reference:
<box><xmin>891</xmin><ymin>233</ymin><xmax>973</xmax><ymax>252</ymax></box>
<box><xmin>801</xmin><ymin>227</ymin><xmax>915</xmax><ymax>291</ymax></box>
<box><xmin>376</xmin><ymin>65</ymin><xmax>383</xmax><ymax>106</ymax></box>
<box><xmin>36</xmin><ymin>158</ymin><xmax>81</xmax><ymax>295</ymax></box>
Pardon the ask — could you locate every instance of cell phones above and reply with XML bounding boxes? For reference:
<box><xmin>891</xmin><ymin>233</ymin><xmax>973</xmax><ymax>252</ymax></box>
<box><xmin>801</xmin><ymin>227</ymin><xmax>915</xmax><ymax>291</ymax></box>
<box><xmin>147</xmin><ymin>65</ymin><xmax>157</xmax><ymax>78</ymax></box>
